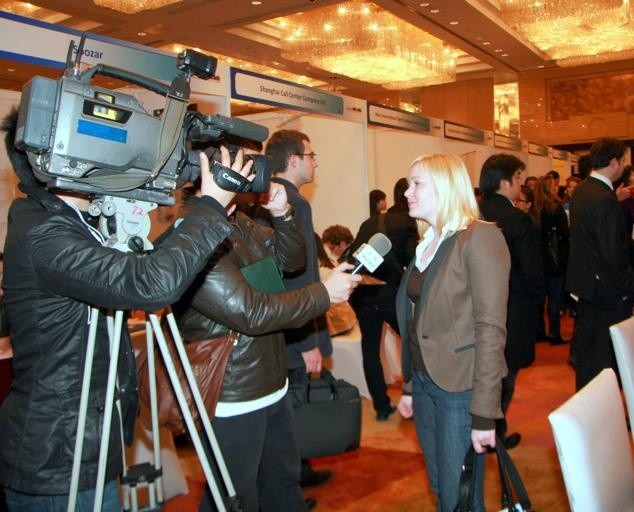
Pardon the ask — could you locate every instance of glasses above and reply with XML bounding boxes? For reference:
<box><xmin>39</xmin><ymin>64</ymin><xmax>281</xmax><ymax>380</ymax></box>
<box><xmin>291</xmin><ymin>152</ymin><xmax>321</xmax><ymax>163</ymax></box>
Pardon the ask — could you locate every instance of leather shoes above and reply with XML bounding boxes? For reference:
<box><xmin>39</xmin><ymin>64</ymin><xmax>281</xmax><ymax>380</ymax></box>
<box><xmin>376</xmin><ymin>404</ymin><xmax>399</xmax><ymax>420</ymax></box>
<box><xmin>535</xmin><ymin>331</ymin><xmax>566</xmax><ymax>346</ymax></box>
<box><xmin>483</xmin><ymin>432</ymin><xmax>521</xmax><ymax>455</ymax></box>
<box><xmin>298</xmin><ymin>464</ymin><xmax>333</xmax><ymax>486</ymax></box>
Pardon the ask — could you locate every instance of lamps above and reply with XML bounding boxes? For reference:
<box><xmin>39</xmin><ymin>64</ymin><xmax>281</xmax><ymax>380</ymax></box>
<box><xmin>497</xmin><ymin>0</ymin><xmax>633</xmax><ymax>71</ymax></box>
<box><xmin>95</xmin><ymin>0</ymin><xmax>178</xmax><ymax>16</ymax></box>
<box><xmin>277</xmin><ymin>0</ymin><xmax>457</xmax><ymax>93</ymax></box>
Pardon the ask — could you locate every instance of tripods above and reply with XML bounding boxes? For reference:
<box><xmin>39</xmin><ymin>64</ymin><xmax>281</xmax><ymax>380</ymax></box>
<box><xmin>67</xmin><ymin>239</ymin><xmax>242</xmax><ymax>512</ymax></box>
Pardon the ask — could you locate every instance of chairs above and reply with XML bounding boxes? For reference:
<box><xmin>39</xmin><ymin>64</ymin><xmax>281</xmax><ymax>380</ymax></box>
<box><xmin>609</xmin><ymin>315</ymin><xmax>634</xmax><ymax>441</ymax></box>
<box><xmin>328</xmin><ymin>324</ymin><xmax>405</xmax><ymax>404</ymax></box>
<box><xmin>127</xmin><ymin>330</ymin><xmax>190</xmax><ymax>507</ymax></box>
<box><xmin>548</xmin><ymin>368</ymin><xmax>633</xmax><ymax>511</ymax></box>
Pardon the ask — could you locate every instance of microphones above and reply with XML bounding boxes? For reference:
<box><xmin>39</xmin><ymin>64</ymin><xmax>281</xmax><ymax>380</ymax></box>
<box><xmin>348</xmin><ymin>232</ymin><xmax>392</xmax><ymax>275</ymax></box>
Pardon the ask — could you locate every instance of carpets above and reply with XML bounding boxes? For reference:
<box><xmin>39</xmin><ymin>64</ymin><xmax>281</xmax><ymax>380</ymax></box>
<box><xmin>125</xmin><ymin>444</ymin><xmax>426</xmax><ymax>512</ymax></box>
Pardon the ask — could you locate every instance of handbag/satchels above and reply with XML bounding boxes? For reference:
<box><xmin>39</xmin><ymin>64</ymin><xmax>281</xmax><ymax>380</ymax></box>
<box><xmin>212</xmin><ymin>256</ymin><xmax>293</xmax><ymax>335</ymax></box>
<box><xmin>129</xmin><ymin>325</ymin><xmax>239</xmax><ymax>439</ymax></box>
<box><xmin>464</xmin><ymin>435</ymin><xmax>534</xmax><ymax>512</ymax></box>
<box><xmin>285</xmin><ymin>363</ymin><xmax>362</xmax><ymax>460</ymax></box>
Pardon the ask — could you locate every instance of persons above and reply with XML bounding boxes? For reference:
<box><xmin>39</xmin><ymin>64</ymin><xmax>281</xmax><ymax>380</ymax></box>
<box><xmin>346</xmin><ymin>137</ymin><xmax>634</xmax><ymax>512</ymax></box>
<box><xmin>0</xmin><ymin>106</ymin><xmax>366</xmax><ymax>512</ymax></box>
